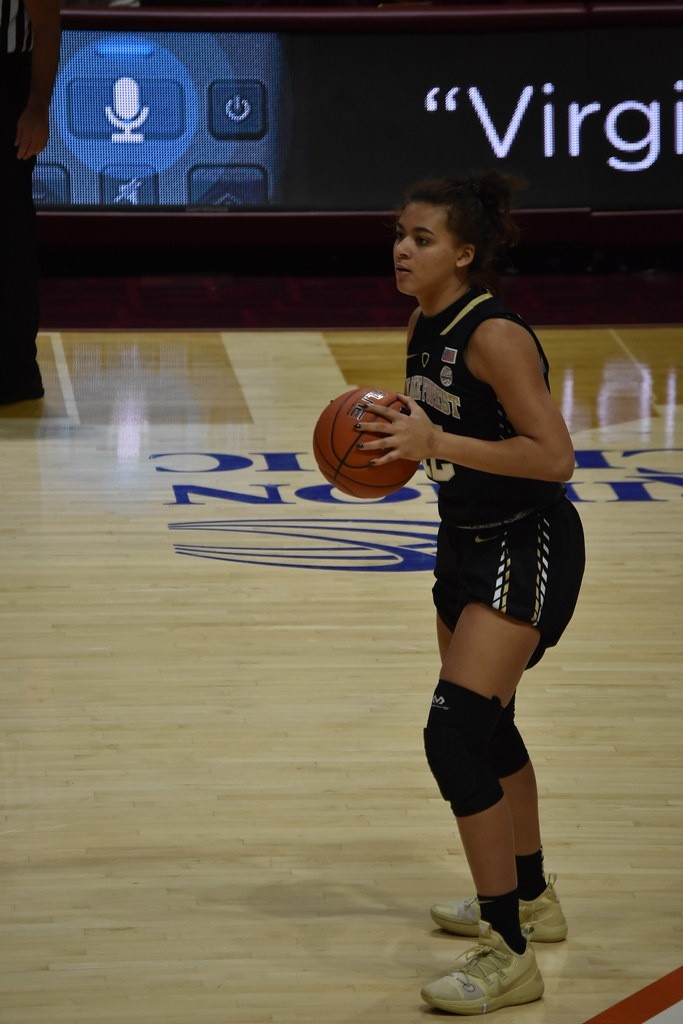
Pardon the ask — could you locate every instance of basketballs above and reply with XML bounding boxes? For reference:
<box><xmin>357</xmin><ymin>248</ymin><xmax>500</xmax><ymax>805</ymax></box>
<box><xmin>313</xmin><ymin>388</ymin><xmax>420</xmax><ymax>500</ymax></box>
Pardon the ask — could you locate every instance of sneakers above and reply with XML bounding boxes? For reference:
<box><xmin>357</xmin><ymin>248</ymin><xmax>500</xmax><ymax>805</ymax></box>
<box><xmin>429</xmin><ymin>874</ymin><xmax>569</xmax><ymax>944</ymax></box>
<box><xmin>415</xmin><ymin>922</ymin><xmax>545</xmax><ymax>1014</ymax></box>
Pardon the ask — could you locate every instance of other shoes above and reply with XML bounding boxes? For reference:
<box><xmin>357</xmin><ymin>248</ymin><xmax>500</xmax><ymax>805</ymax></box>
<box><xmin>0</xmin><ymin>364</ymin><xmax>44</xmax><ymax>406</ymax></box>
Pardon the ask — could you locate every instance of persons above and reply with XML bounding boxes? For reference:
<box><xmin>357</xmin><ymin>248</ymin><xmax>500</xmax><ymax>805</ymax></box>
<box><xmin>352</xmin><ymin>176</ymin><xmax>587</xmax><ymax>1014</ymax></box>
<box><xmin>0</xmin><ymin>0</ymin><xmax>61</xmax><ymax>408</ymax></box>
